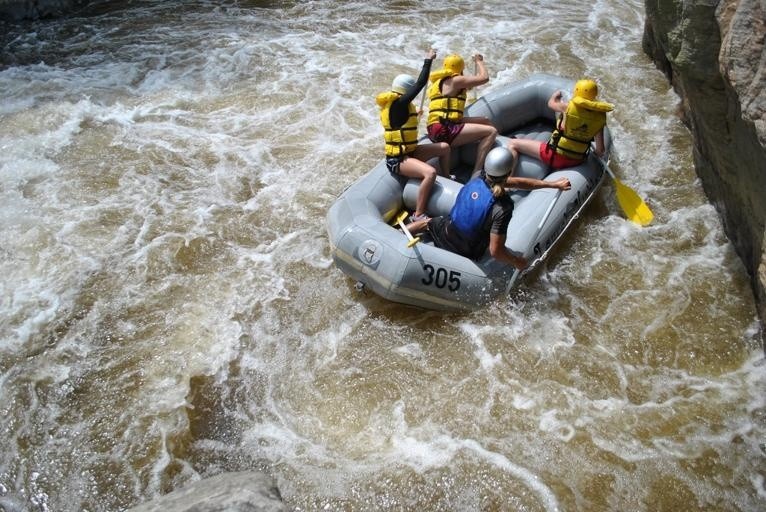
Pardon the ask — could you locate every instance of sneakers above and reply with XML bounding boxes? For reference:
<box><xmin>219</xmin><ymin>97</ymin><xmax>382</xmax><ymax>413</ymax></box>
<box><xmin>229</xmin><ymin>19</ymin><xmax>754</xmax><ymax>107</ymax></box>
<box><xmin>448</xmin><ymin>174</ymin><xmax>464</xmax><ymax>183</ymax></box>
<box><xmin>409</xmin><ymin>212</ymin><xmax>430</xmax><ymax>222</ymax></box>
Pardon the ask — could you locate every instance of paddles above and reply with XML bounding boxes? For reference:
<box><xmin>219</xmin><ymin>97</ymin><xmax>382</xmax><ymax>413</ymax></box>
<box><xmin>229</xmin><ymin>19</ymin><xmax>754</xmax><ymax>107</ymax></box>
<box><xmin>468</xmin><ymin>56</ymin><xmax>480</xmax><ymax>104</ymax></box>
<box><xmin>555</xmin><ymin>92</ymin><xmax>654</xmax><ymax>227</ymax></box>
<box><xmin>391</xmin><ymin>208</ymin><xmax>420</xmax><ymax>248</ymax></box>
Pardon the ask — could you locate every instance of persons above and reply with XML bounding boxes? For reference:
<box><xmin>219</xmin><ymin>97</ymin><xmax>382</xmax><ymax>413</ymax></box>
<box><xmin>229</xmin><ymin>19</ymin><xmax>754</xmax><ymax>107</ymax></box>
<box><xmin>396</xmin><ymin>144</ymin><xmax>573</xmax><ymax>273</ymax></box>
<box><xmin>502</xmin><ymin>78</ymin><xmax>618</xmax><ymax>193</ymax></box>
<box><xmin>374</xmin><ymin>49</ymin><xmax>457</xmax><ymax>224</ymax></box>
<box><xmin>425</xmin><ymin>50</ymin><xmax>498</xmax><ymax>177</ymax></box>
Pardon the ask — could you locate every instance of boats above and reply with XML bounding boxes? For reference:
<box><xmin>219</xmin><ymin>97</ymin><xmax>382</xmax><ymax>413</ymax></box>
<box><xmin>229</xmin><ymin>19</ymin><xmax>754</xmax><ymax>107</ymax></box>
<box><xmin>325</xmin><ymin>74</ymin><xmax>612</xmax><ymax>315</ymax></box>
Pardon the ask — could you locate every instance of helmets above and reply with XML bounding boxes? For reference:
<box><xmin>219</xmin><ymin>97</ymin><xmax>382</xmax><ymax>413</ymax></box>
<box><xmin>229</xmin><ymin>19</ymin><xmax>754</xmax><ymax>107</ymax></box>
<box><xmin>574</xmin><ymin>79</ymin><xmax>598</xmax><ymax>100</ymax></box>
<box><xmin>443</xmin><ymin>54</ymin><xmax>465</xmax><ymax>74</ymax></box>
<box><xmin>484</xmin><ymin>146</ymin><xmax>513</xmax><ymax>177</ymax></box>
<box><xmin>392</xmin><ymin>74</ymin><xmax>416</xmax><ymax>95</ymax></box>
